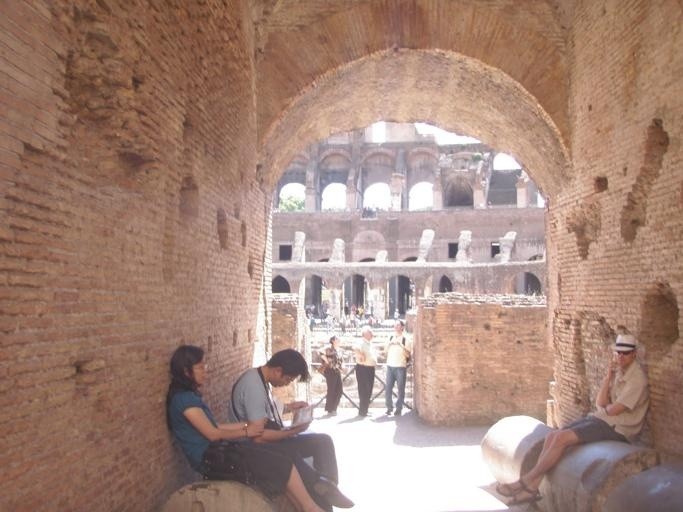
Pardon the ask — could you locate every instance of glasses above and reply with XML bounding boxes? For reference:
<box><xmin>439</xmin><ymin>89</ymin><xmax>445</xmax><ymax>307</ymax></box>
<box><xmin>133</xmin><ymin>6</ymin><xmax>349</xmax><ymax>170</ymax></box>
<box><xmin>192</xmin><ymin>363</ymin><xmax>209</xmax><ymax>371</ymax></box>
<box><xmin>615</xmin><ymin>351</ymin><xmax>634</xmax><ymax>355</ymax></box>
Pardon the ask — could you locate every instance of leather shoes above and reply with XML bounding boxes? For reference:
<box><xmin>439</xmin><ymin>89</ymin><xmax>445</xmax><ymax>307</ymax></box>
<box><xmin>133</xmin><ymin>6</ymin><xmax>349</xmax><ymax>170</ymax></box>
<box><xmin>313</xmin><ymin>476</ymin><xmax>355</xmax><ymax>508</ymax></box>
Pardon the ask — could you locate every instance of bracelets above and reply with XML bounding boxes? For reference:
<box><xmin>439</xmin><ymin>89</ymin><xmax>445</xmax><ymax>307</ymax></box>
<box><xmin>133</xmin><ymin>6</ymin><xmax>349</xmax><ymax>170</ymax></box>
<box><xmin>243</xmin><ymin>425</ymin><xmax>247</xmax><ymax>438</ymax></box>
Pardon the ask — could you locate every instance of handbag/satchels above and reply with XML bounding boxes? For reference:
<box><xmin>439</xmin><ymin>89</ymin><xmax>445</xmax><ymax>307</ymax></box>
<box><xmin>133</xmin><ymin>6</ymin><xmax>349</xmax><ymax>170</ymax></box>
<box><xmin>318</xmin><ymin>364</ymin><xmax>328</xmax><ymax>377</ymax></box>
<box><xmin>208</xmin><ymin>442</ymin><xmax>253</xmax><ymax>484</ymax></box>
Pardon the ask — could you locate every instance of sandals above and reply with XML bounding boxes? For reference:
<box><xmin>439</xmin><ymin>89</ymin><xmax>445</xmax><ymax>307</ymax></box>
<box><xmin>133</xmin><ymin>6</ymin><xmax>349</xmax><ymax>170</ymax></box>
<box><xmin>496</xmin><ymin>479</ymin><xmax>541</xmax><ymax>506</ymax></box>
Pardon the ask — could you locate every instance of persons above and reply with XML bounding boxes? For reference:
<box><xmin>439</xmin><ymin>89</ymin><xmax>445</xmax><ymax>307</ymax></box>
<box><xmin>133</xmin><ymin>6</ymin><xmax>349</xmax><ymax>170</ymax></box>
<box><xmin>227</xmin><ymin>349</ymin><xmax>354</xmax><ymax>512</ymax></box>
<box><xmin>167</xmin><ymin>345</ymin><xmax>325</xmax><ymax>512</ymax></box>
<box><xmin>494</xmin><ymin>333</ymin><xmax>650</xmax><ymax>506</ymax></box>
<box><xmin>321</xmin><ymin>336</ymin><xmax>347</xmax><ymax>416</ymax></box>
<box><xmin>306</xmin><ymin>299</ymin><xmax>400</xmax><ymax>335</ymax></box>
<box><xmin>384</xmin><ymin>319</ymin><xmax>412</xmax><ymax>417</ymax></box>
<box><xmin>352</xmin><ymin>326</ymin><xmax>377</xmax><ymax>416</ymax></box>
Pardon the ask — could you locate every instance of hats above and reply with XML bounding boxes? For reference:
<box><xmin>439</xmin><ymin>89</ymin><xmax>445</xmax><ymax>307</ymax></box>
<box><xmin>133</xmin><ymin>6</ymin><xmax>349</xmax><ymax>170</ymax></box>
<box><xmin>610</xmin><ymin>334</ymin><xmax>636</xmax><ymax>352</ymax></box>
